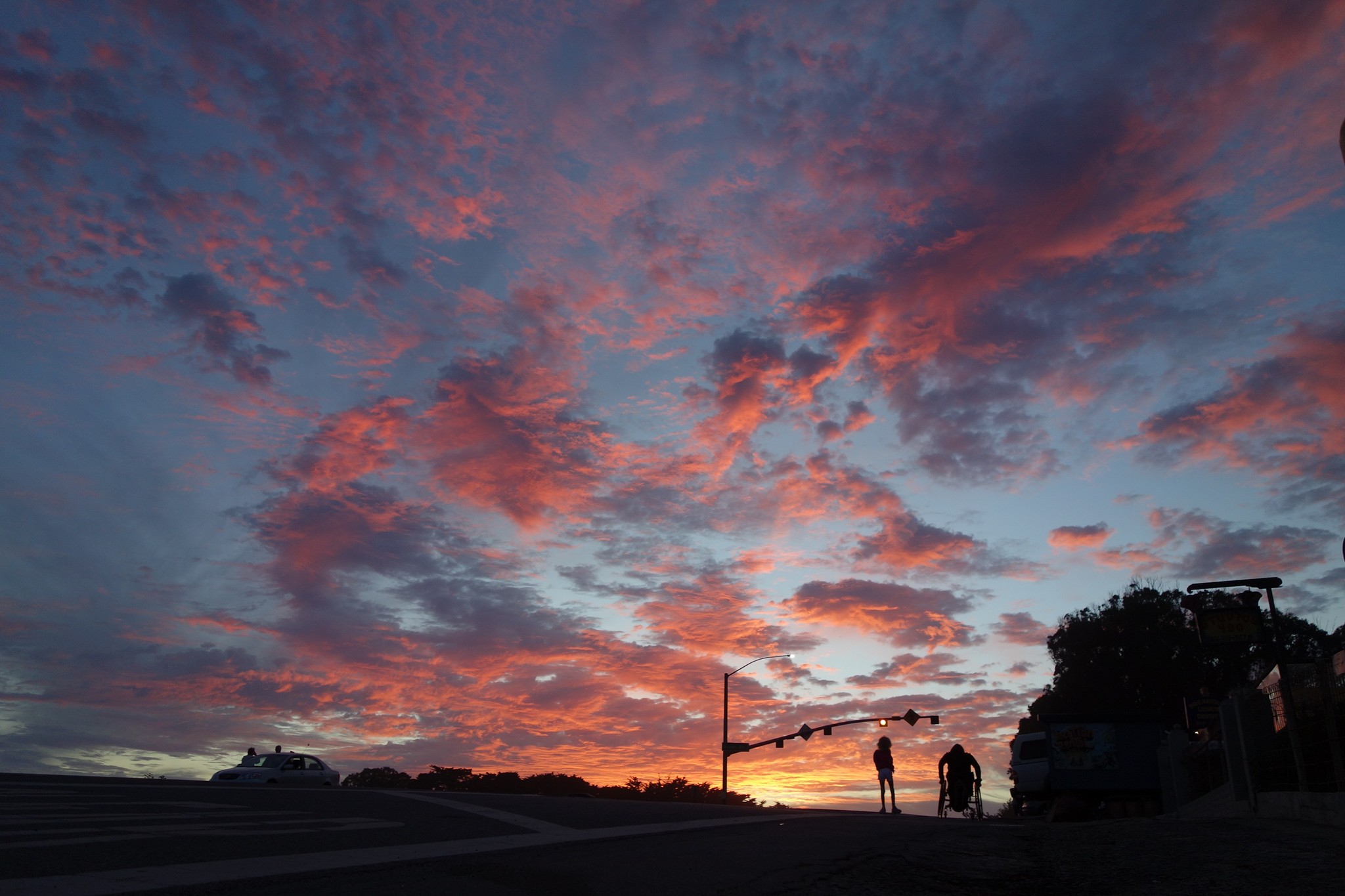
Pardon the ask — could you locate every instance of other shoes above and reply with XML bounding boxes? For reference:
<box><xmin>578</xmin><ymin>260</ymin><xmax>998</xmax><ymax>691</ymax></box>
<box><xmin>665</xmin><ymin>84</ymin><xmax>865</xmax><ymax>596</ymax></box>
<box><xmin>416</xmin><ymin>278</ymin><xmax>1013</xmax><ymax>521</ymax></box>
<box><xmin>879</xmin><ymin>808</ymin><xmax>886</xmax><ymax>814</ymax></box>
<box><xmin>892</xmin><ymin>807</ymin><xmax>901</xmax><ymax>814</ymax></box>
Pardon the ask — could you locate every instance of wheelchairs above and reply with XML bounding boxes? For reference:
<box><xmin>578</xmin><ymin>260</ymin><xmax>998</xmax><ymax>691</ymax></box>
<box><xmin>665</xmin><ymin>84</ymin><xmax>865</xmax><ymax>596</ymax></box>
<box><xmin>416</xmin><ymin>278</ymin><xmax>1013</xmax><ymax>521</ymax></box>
<box><xmin>938</xmin><ymin>778</ymin><xmax>984</xmax><ymax>820</ymax></box>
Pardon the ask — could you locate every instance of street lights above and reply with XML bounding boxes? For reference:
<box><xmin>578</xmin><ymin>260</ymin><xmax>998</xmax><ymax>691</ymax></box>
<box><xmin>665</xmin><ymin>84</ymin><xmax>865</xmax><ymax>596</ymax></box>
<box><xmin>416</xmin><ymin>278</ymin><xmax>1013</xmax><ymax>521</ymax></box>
<box><xmin>721</xmin><ymin>654</ymin><xmax>796</xmax><ymax>794</ymax></box>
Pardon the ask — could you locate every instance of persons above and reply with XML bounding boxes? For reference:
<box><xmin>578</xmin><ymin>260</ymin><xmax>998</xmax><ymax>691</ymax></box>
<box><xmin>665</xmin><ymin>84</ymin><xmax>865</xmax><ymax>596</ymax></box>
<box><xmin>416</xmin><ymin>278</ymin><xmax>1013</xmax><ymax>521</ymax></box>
<box><xmin>938</xmin><ymin>744</ymin><xmax>982</xmax><ymax>788</ymax></box>
<box><xmin>241</xmin><ymin>747</ymin><xmax>260</xmax><ymax>766</ymax></box>
<box><xmin>872</xmin><ymin>735</ymin><xmax>902</xmax><ymax>814</ymax></box>
<box><xmin>275</xmin><ymin>745</ymin><xmax>281</xmax><ymax>753</ymax></box>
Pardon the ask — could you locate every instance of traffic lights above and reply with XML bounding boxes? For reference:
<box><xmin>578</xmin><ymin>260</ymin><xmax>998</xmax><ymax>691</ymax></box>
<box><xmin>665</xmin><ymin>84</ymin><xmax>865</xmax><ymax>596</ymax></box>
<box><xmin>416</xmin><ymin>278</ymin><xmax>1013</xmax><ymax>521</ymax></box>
<box><xmin>879</xmin><ymin>719</ymin><xmax>888</xmax><ymax>727</ymax></box>
